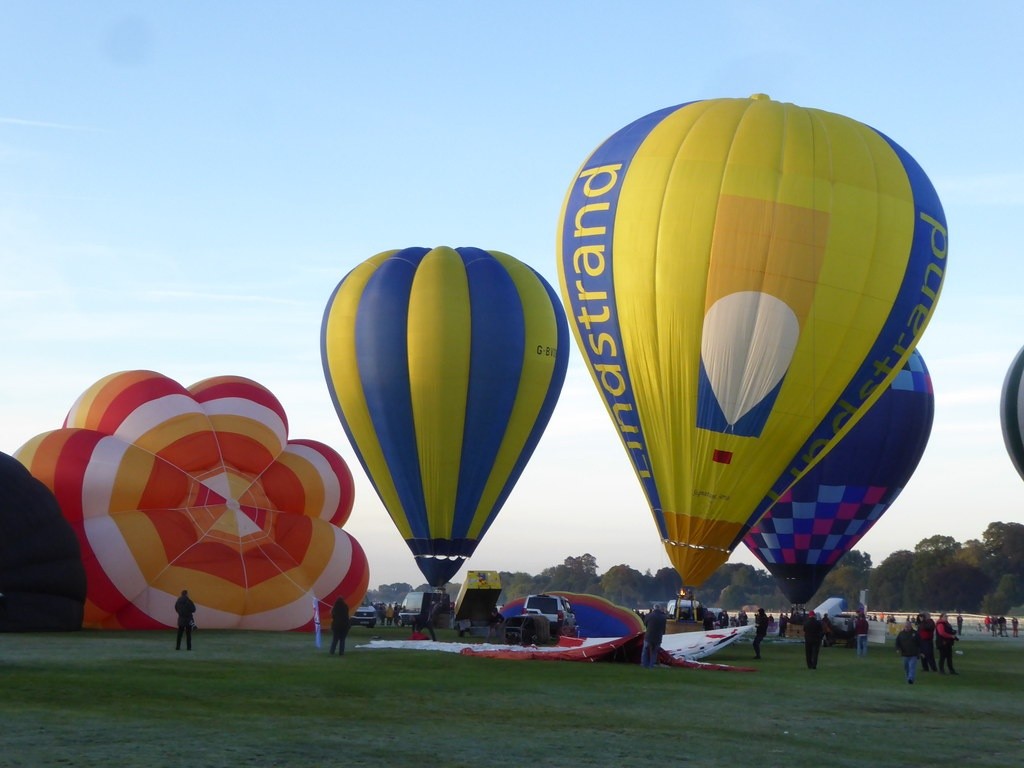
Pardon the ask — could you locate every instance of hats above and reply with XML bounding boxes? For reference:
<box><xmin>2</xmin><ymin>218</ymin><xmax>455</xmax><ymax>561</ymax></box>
<box><xmin>809</xmin><ymin>610</ymin><xmax>815</xmax><ymax>617</ymax></box>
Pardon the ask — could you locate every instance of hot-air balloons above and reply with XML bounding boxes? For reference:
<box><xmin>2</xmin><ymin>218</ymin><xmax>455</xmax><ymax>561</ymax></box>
<box><xmin>555</xmin><ymin>90</ymin><xmax>951</xmax><ymax>633</ymax></box>
<box><xmin>319</xmin><ymin>246</ymin><xmax>570</xmax><ymax>595</ymax></box>
<box><xmin>740</xmin><ymin>341</ymin><xmax>937</xmax><ymax>643</ymax></box>
<box><xmin>9</xmin><ymin>367</ymin><xmax>372</xmax><ymax>631</ymax></box>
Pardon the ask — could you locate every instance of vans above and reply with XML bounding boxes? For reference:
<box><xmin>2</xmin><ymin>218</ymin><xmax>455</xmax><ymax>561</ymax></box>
<box><xmin>648</xmin><ymin>598</ymin><xmax>725</xmax><ymax>623</ymax></box>
<box><xmin>350</xmin><ymin>596</ymin><xmax>378</xmax><ymax>629</ymax></box>
<box><xmin>396</xmin><ymin>591</ymin><xmax>452</xmax><ymax>630</ymax></box>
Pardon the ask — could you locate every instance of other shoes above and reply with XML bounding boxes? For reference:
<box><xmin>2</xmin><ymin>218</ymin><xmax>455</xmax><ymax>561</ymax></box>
<box><xmin>808</xmin><ymin>665</ymin><xmax>815</xmax><ymax>669</ymax></box>
<box><xmin>908</xmin><ymin>679</ymin><xmax>913</xmax><ymax>684</ymax></box>
<box><xmin>753</xmin><ymin>656</ymin><xmax>761</xmax><ymax>659</ymax></box>
<box><xmin>951</xmin><ymin>671</ymin><xmax>960</xmax><ymax>675</ymax></box>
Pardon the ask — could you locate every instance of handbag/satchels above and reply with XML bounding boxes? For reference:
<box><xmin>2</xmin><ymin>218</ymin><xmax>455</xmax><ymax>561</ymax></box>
<box><xmin>190</xmin><ymin>623</ymin><xmax>198</xmax><ymax>632</ymax></box>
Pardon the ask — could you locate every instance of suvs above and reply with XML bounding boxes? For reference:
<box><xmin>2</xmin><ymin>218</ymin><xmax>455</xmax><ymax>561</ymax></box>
<box><xmin>522</xmin><ymin>593</ymin><xmax>578</xmax><ymax>638</ymax></box>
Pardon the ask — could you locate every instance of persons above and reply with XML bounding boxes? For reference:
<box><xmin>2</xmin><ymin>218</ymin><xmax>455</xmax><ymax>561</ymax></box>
<box><xmin>634</xmin><ymin>604</ymin><xmax>1019</xmax><ymax>682</ymax></box>
<box><xmin>175</xmin><ymin>590</ymin><xmax>196</xmax><ymax>650</ymax></box>
<box><xmin>485</xmin><ymin>609</ymin><xmax>507</xmax><ymax>644</ymax></box>
<box><xmin>329</xmin><ymin>595</ymin><xmax>349</xmax><ymax>656</ymax></box>
<box><xmin>371</xmin><ymin>602</ymin><xmax>403</xmax><ymax>625</ymax></box>
